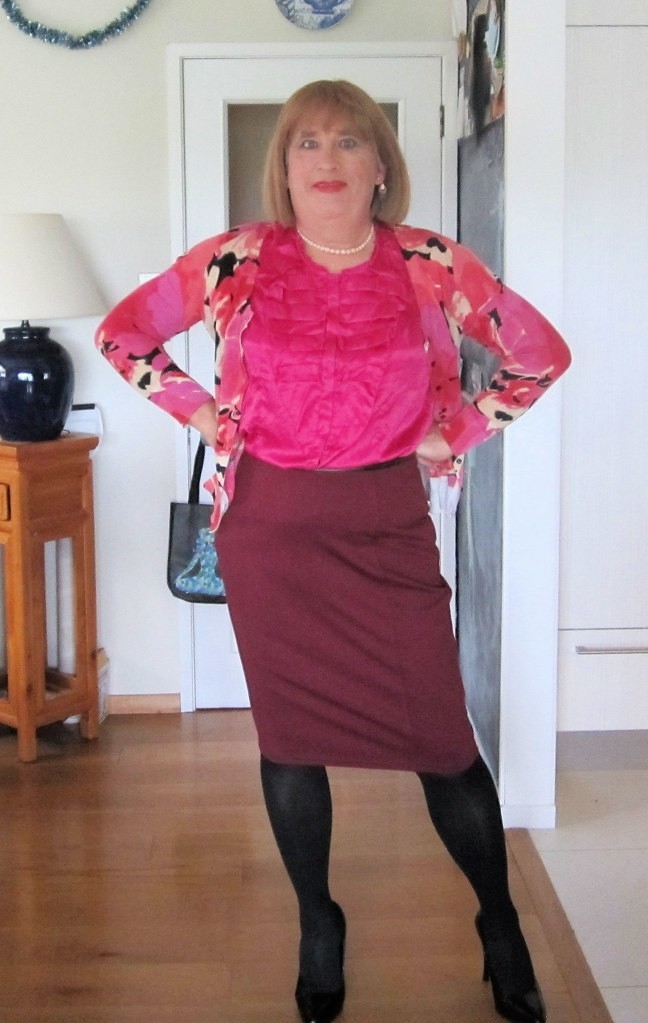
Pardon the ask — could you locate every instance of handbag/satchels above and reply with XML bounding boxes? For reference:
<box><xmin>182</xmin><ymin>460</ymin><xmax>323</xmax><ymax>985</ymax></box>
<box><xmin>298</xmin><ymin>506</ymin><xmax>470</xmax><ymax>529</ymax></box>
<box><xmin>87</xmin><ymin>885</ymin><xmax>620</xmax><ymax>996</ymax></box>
<box><xmin>168</xmin><ymin>502</ymin><xmax>233</xmax><ymax>606</ymax></box>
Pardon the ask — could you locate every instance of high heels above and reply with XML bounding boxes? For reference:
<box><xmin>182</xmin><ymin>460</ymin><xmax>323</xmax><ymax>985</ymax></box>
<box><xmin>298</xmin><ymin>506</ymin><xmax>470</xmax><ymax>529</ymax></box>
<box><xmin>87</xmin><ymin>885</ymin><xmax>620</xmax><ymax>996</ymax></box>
<box><xmin>474</xmin><ymin>906</ymin><xmax>548</xmax><ymax>1023</ymax></box>
<box><xmin>294</xmin><ymin>900</ymin><xmax>347</xmax><ymax>1023</ymax></box>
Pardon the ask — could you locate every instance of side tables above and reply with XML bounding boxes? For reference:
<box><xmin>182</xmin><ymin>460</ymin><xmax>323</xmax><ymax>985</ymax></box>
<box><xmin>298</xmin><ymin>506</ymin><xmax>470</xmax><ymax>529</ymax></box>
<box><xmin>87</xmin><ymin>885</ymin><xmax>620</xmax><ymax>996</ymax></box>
<box><xmin>0</xmin><ymin>432</ymin><xmax>100</xmax><ymax>762</ymax></box>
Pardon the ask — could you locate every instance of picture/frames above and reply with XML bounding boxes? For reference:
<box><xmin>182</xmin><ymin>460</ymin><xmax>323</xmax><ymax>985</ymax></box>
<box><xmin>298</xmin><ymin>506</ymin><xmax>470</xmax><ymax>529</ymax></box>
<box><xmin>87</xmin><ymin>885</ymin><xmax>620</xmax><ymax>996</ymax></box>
<box><xmin>450</xmin><ymin>0</ymin><xmax>507</xmax><ymax>142</ymax></box>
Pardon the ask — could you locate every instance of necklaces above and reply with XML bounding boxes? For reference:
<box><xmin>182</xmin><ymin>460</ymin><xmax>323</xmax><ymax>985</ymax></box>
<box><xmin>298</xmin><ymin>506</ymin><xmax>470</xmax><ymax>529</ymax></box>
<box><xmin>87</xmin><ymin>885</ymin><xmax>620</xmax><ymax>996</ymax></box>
<box><xmin>293</xmin><ymin>220</ymin><xmax>375</xmax><ymax>256</ymax></box>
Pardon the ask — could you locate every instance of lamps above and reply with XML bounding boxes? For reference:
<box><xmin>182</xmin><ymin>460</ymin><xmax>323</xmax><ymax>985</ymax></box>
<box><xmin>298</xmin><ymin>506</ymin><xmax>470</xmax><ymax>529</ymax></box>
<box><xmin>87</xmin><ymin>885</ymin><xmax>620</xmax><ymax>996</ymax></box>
<box><xmin>0</xmin><ymin>213</ymin><xmax>108</xmax><ymax>443</ymax></box>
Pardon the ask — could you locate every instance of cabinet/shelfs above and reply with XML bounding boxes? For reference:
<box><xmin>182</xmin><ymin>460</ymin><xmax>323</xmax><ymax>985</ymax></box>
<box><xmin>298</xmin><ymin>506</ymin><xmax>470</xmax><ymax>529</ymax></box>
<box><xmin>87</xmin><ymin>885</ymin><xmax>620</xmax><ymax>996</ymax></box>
<box><xmin>555</xmin><ymin>23</ymin><xmax>647</xmax><ymax>734</ymax></box>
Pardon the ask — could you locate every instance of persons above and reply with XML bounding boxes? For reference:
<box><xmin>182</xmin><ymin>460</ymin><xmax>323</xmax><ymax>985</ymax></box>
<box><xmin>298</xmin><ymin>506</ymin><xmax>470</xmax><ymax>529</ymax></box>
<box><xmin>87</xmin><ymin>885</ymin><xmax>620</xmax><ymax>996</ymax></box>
<box><xmin>90</xmin><ymin>73</ymin><xmax>573</xmax><ymax>1023</ymax></box>
